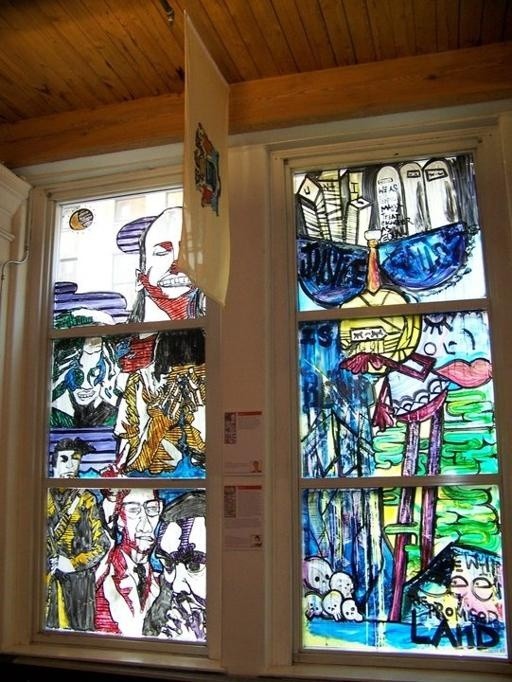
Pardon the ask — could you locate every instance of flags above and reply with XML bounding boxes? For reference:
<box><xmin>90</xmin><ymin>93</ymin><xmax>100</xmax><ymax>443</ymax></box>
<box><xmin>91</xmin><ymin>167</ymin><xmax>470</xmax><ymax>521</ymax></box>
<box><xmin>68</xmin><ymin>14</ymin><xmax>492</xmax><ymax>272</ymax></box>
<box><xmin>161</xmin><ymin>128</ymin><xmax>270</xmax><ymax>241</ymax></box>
<box><xmin>175</xmin><ymin>8</ymin><xmax>233</xmax><ymax>307</ymax></box>
